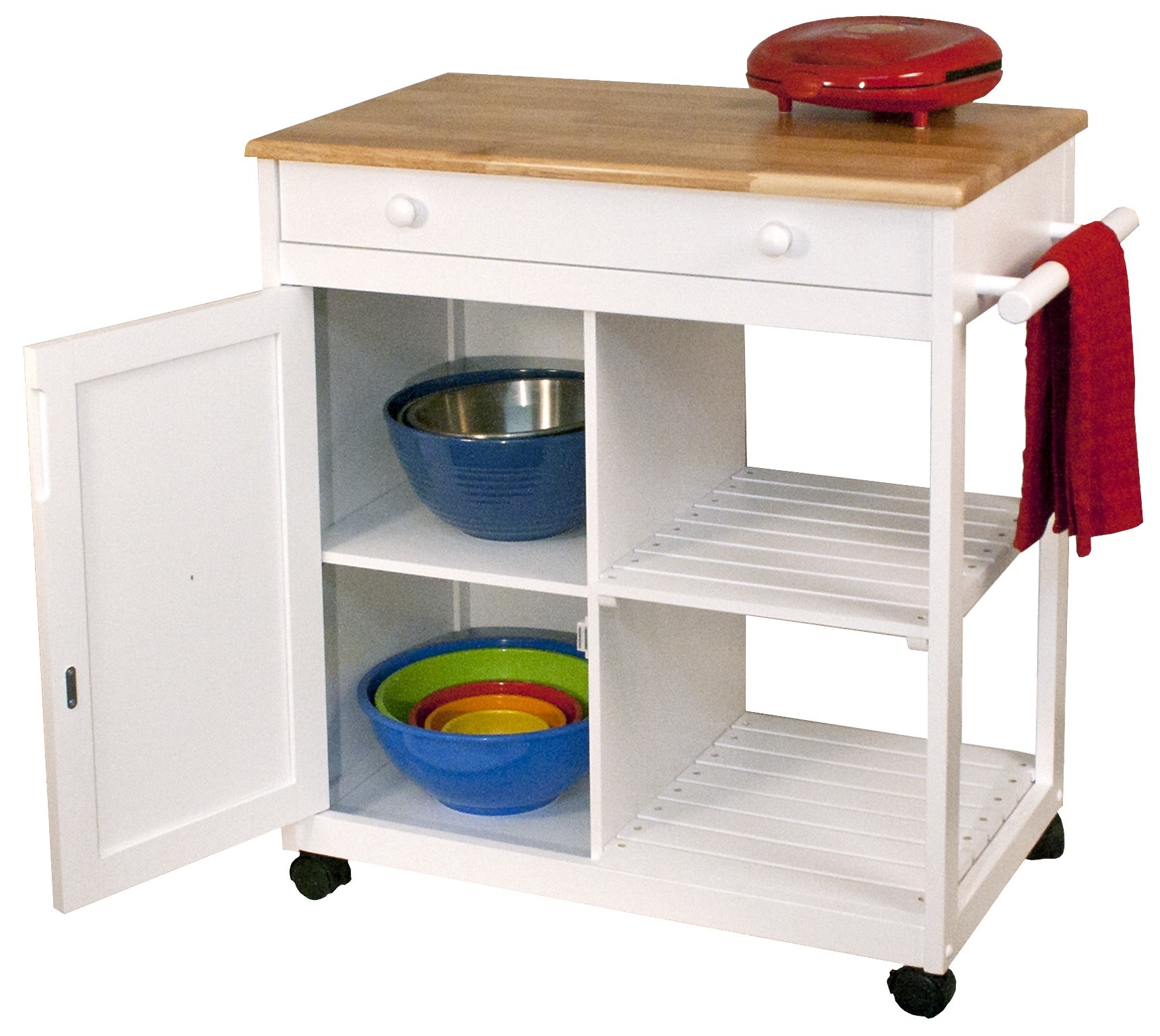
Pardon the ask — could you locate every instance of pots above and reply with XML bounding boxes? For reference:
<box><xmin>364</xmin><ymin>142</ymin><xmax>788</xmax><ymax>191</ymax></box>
<box><xmin>745</xmin><ymin>16</ymin><xmax>1003</xmax><ymax>130</ymax></box>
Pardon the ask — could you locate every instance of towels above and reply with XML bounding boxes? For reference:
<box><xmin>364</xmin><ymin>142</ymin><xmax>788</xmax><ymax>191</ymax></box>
<box><xmin>1011</xmin><ymin>218</ymin><xmax>1146</xmax><ymax>561</ymax></box>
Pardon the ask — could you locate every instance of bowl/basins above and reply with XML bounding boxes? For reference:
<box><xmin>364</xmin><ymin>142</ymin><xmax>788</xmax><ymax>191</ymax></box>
<box><xmin>383</xmin><ymin>368</ymin><xmax>585</xmax><ymax>544</ymax></box>
<box><xmin>357</xmin><ymin>635</ymin><xmax>590</xmax><ymax>816</ymax></box>
